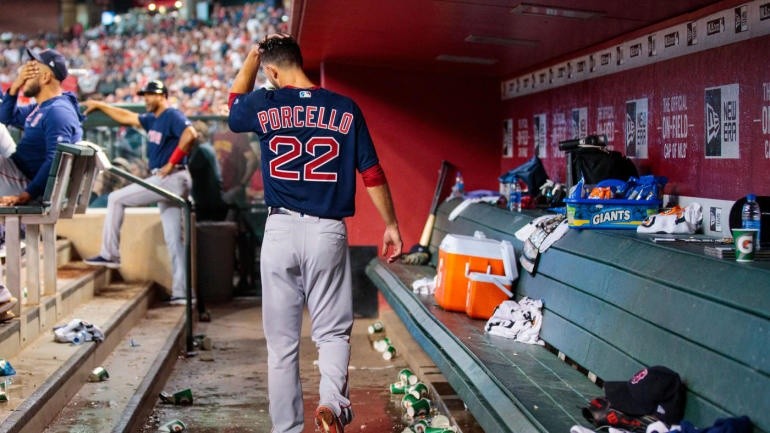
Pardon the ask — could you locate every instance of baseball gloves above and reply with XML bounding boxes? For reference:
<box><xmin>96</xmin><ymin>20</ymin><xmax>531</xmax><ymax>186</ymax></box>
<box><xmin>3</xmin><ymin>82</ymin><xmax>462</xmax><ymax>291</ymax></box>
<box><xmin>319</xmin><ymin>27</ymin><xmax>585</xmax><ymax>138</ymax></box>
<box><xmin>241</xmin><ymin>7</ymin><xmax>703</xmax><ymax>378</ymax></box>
<box><xmin>581</xmin><ymin>395</ymin><xmax>673</xmax><ymax>433</ymax></box>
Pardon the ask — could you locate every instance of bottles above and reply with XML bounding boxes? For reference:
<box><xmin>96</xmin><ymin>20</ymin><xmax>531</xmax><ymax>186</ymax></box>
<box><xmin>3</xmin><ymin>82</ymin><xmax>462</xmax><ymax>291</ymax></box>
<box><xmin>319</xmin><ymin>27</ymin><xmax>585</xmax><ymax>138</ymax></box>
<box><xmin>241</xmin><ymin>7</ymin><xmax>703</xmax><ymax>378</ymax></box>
<box><xmin>741</xmin><ymin>194</ymin><xmax>760</xmax><ymax>251</ymax></box>
<box><xmin>455</xmin><ymin>171</ymin><xmax>463</xmax><ymax>195</ymax></box>
<box><xmin>509</xmin><ymin>177</ymin><xmax>522</xmax><ymax>213</ymax></box>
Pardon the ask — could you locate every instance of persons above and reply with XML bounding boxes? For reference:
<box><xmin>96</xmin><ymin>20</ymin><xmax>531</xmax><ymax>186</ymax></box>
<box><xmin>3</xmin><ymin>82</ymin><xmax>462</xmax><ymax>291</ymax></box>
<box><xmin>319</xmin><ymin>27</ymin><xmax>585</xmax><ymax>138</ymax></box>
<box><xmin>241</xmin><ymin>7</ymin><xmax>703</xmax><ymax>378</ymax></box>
<box><xmin>229</xmin><ymin>35</ymin><xmax>403</xmax><ymax>433</ymax></box>
<box><xmin>208</xmin><ymin>103</ymin><xmax>262</xmax><ymax>282</ymax></box>
<box><xmin>0</xmin><ymin>46</ymin><xmax>87</xmax><ymax>314</ymax></box>
<box><xmin>185</xmin><ymin>126</ymin><xmax>230</xmax><ymax>223</ymax></box>
<box><xmin>77</xmin><ymin>81</ymin><xmax>199</xmax><ymax>305</ymax></box>
<box><xmin>0</xmin><ymin>0</ymin><xmax>291</xmax><ymax>207</ymax></box>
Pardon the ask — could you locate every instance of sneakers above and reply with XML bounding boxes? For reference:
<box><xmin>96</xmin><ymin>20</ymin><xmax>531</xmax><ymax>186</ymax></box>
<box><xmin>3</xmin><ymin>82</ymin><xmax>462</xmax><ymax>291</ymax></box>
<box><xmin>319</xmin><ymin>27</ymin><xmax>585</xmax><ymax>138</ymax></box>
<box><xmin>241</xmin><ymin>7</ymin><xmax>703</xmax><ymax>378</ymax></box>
<box><xmin>315</xmin><ymin>405</ymin><xmax>343</xmax><ymax>433</ymax></box>
<box><xmin>84</xmin><ymin>255</ymin><xmax>121</xmax><ymax>269</ymax></box>
<box><xmin>165</xmin><ymin>298</ymin><xmax>187</xmax><ymax>305</ymax></box>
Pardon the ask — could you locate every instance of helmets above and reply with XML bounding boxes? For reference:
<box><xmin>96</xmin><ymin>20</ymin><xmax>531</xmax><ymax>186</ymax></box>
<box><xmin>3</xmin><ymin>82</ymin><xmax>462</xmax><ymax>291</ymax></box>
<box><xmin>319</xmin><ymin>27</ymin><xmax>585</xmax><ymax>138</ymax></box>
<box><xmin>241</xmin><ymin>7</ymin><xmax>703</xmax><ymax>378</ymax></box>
<box><xmin>136</xmin><ymin>80</ymin><xmax>168</xmax><ymax>99</ymax></box>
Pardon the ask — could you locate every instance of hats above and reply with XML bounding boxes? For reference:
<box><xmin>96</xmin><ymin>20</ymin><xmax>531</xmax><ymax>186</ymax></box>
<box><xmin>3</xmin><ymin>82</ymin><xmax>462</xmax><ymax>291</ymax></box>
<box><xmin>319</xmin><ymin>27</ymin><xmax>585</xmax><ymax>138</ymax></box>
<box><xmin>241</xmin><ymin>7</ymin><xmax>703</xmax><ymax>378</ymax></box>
<box><xmin>604</xmin><ymin>366</ymin><xmax>686</xmax><ymax>425</ymax></box>
<box><xmin>26</xmin><ymin>47</ymin><xmax>68</xmax><ymax>81</ymax></box>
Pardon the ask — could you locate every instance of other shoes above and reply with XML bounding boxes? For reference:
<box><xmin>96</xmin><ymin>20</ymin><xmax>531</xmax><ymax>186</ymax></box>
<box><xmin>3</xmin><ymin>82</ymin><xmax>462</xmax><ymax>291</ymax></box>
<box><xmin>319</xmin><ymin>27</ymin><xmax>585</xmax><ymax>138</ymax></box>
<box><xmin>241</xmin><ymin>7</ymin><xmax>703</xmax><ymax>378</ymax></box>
<box><xmin>0</xmin><ymin>288</ymin><xmax>18</xmax><ymax>321</ymax></box>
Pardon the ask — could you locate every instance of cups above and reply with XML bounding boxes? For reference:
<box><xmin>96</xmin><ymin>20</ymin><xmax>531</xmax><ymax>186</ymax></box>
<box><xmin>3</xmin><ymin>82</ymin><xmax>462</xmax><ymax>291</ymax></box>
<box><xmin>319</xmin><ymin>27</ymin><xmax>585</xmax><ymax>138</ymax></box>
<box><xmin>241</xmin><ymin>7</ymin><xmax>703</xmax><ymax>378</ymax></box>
<box><xmin>0</xmin><ymin>380</ymin><xmax>10</xmax><ymax>402</ymax></box>
<box><xmin>390</xmin><ymin>369</ymin><xmax>457</xmax><ymax>433</ymax></box>
<box><xmin>732</xmin><ymin>228</ymin><xmax>759</xmax><ymax>262</ymax></box>
<box><xmin>373</xmin><ymin>337</ymin><xmax>396</xmax><ymax>360</ymax></box>
<box><xmin>368</xmin><ymin>320</ymin><xmax>384</xmax><ymax>334</ymax></box>
<box><xmin>88</xmin><ymin>366</ymin><xmax>109</xmax><ymax>382</ymax></box>
<box><xmin>158</xmin><ymin>420</ymin><xmax>186</xmax><ymax>433</ymax></box>
<box><xmin>172</xmin><ymin>388</ymin><xmax>193</xmax><ymax>406</ymax></box>
<box><xmin>193</xmin><ymin>333</ymin><xmax>212</xmax><ymax>350</ymax></box>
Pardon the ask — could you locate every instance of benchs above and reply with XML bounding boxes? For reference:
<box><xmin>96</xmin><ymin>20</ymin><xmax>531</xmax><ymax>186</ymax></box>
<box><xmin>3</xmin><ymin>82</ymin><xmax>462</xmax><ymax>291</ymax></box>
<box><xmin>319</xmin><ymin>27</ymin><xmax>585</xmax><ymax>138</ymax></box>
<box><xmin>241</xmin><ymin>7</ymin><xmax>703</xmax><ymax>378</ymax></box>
<box><xmin>366</xmin><ymin>195</ymin><xmax>770</xmax><ymax>433</ymax></box>
<box><xmin>0</xmin><ymin>142</ymin><xmax>99</xmax><ymax>319</ymax></box>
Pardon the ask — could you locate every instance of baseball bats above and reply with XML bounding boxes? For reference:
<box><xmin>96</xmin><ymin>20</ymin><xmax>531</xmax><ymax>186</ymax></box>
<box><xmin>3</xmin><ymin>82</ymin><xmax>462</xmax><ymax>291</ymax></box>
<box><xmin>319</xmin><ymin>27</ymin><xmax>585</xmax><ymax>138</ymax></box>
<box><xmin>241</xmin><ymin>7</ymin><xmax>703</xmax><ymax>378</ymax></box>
<box><xmin>416</xmin><ymin>160</ymin><xmax>449</xmax><ymax>250</ymax></box>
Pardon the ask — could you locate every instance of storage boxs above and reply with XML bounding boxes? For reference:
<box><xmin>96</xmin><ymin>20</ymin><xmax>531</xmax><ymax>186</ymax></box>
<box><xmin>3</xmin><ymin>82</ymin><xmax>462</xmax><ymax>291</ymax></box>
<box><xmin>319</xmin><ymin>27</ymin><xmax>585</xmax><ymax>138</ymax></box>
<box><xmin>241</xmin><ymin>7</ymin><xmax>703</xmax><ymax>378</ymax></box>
<box><xmin>434</xmin><ymin>228</ymin><xmax>519</xmax><ymax>322</ymax></box>
<box><xmin>562</xmin><ymin>197</ymin><xmax>661</xmax><ymax>230</ymax></box>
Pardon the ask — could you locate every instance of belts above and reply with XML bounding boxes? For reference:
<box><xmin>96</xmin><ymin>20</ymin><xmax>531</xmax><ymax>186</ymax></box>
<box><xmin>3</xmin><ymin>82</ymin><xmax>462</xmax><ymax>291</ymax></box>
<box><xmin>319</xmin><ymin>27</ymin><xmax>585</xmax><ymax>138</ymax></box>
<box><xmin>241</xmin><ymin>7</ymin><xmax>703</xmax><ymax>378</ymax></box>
<box><xmin>269</xmin><ymin>206</ymin><xmax>296</xmax><ymax>215</ymax></box>
